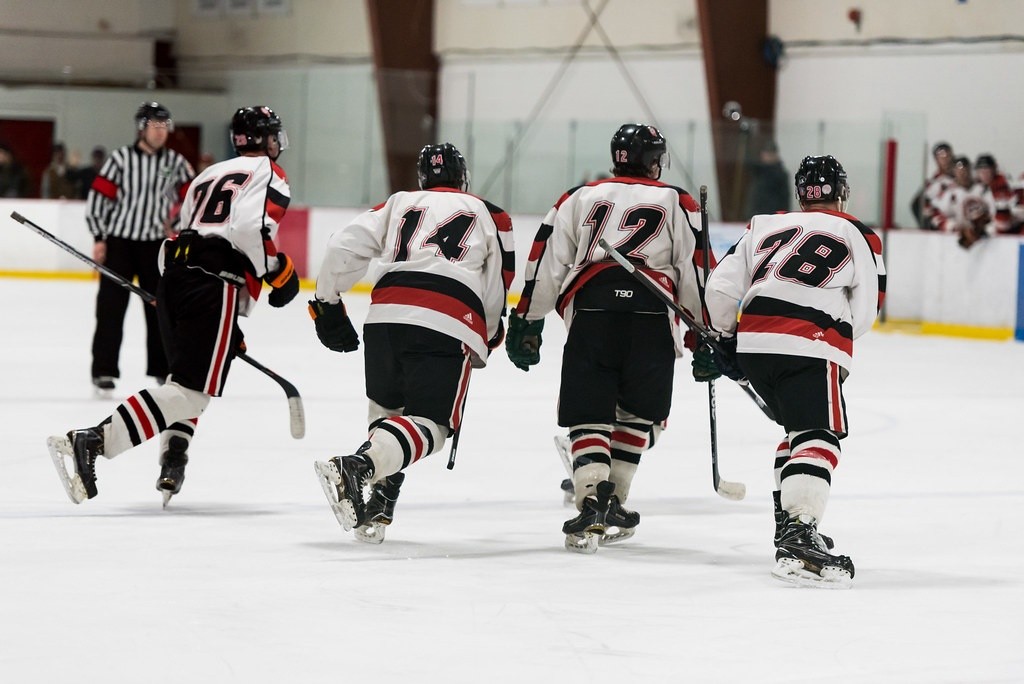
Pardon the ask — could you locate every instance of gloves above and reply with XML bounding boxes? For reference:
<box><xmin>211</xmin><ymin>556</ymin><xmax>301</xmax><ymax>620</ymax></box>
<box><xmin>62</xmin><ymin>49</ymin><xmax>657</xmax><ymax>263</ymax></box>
<box><xmin>505</xmin><ymin>309</ymin><xmax>545</xmax><ymax>371</ymax></box>
<box><xmin>691</xmin><ymin>335</ymin><xmax>722</xmax><ymax>382</ymax></box>
<box><xmin>487</xmin><ymin>318</ymin><xmax>505</xmax><ymax>358</ymax></box>
<box><xmin>308</xmin><ymin>292</ymin><xmax>360</xmax><ymax>352</ymax></box>
<box><xmin>263</xmin><ymin>251</ymin><xmax>300</xmax><ymax>307</ymax></box>
<box><xmin>717</xmin><ymin>334</ymin><xmax>750</xmax><ymax>388</ymax></box>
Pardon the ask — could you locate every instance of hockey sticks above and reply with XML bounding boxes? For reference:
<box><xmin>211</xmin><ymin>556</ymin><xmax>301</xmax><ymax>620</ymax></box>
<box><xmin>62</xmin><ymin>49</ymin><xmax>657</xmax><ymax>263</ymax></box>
<box><xmin>448</xmin><ymin>396</ymin><xmax>467</xmax><ymax>470</ymax></box>
<box><xmin>9</xmin><ymin>210</ymin><xmax>307</xmax><ymax>440</ymax></box>
<box><xmin>596</xmin><ymin>187</ymin><xmax>777</xmax><ymax>503</ymax></box>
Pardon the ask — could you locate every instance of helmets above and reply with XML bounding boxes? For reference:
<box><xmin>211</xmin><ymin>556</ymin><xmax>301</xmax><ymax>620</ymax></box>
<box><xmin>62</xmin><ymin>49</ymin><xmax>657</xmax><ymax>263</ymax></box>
<box><xmin>933</xmin><ymin>144</ymin><xmax>951</xmax><ymax>156</ymax></box>
<box><xmin>227</xmin><ymin>105</ymin><xmax>282</xmax><ymax>155</ymax></box>
<box><xmin>974</xmin><ymin>154</ymin><xmax>997</xmax><ymax>170</ymax></box>
<box><xmin>794</xmin><ymin>155</ymin><xmax>849</xmax><ymax>205</ymax></box>
<box><xmin>950</xmin><ymin>158</ymin><xmax>971</xmax><ymax>170</ymax></box>
<box><xmin>135</xmin><ymin>101</ymin><xmax>173</xmax><ymax>132</ymax></box>
<box><xmin>611</xmin><ymin>123</ymin><xmax>666</xmax><ymax>177</ymax></box>
<box><xmin>417</xmin><ymin>142</ymin><xmax>469</xmax><ymax>190</ymax></box>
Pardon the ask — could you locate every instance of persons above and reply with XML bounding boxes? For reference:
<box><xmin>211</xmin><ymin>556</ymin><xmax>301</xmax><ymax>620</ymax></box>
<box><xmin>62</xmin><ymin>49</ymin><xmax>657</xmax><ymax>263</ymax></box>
<box><xmin>42</xmin><ymin>142</ymin><xmax>73</xmax><ymax>199</ymax></box>
<box><xmin>705</xmin><ymin>155</ymin><xmax>887</xmax><ymax>589</ymax></box>
<box><xmin>45</xmin><ymin>106</ymin><xmax>300</xmax><ymax>510</ymax></box>
<box><xmin>307</xmin><ymin>142</ymin><xmax>515</xmax><ymax>544</ymax></box>
<box><xmin>85</xmin><ymin>101</ymin><xmax>195</xmax><ymax>399</ymax></box>
<box><xmin>505</xmin><ymin>123</ymin><xmax>717</xmax><ymax>553</ymax></box>
<box><xmin>910</xmin><ymin>143</ymin><xmax>1024</xmax><ymax>248</ymax></box>
<box><xmin>0</xmin><ymin>144</ymin><xmax>35</xmax><ymax>198</ymax></box>
<box><xmin>78</xmin><ymin>144</ymin><xmax>105</xmax><ymax>200</ymax></box>
<box><xmin>743</xmin><ymin>142</ymin><xmax>791</xmax><ymax>221</ymax></box>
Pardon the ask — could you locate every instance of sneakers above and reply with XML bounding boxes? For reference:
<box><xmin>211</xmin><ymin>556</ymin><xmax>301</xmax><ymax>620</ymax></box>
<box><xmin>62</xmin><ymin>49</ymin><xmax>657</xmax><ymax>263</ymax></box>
<box><xmin>92</xmin><ymin>377</ymin><xmax>115</xmax><ymax>398</ymax></box>
<box><xmin>562</xmin><ymin>480</ymin><xmax>615</xmax><ymax>554</ymax></box>
<box><xmin>47</xmin><ymin>415</ymin><xmax>113</xmax><ymax>504</ymax></box>
<box><xmin>156</xmin><ymin>436</ymin><xmax>189</xmax><ymax>509</ymax></box>
<box><xmin>773</xmin><ymin>490</ymin><xmax>834</xmax><ymax>554</ymax></box>
<box><xmin>772</xmin><ymin>509</ymin><xmax>855</xmax><ymax>590</ymax></box>
<box><xmin>314</xmin><ymin>441</ymin><xmax>376</xmax><ymax>532</ymax></box>
<box><xmin>599</xmin><ymin>494</ymin><xmax>641</xmax><ymax>547</ymax></box>
<box><xmin>354</xmin><ymin>471</ymin><xmax>405</xmax><ymax>544</ymax></box>
<box><xmin>561</xmin><ymin>477</ymin><xmax>577</xmax><ymax>506</ymax></box>
